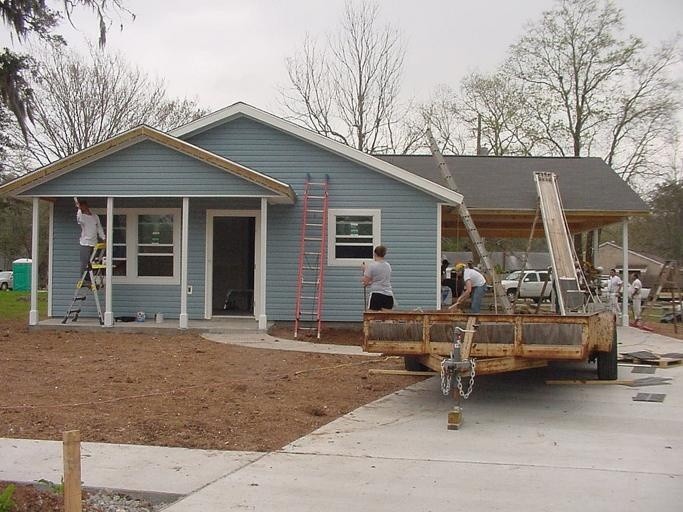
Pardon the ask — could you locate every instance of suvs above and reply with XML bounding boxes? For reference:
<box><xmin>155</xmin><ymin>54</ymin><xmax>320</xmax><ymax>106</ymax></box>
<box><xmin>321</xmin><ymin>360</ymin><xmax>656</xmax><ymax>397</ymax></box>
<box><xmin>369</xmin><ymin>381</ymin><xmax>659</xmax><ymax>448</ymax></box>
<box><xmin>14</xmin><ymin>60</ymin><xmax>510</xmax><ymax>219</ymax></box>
<box><xmin>498</xmin><ymin>268</ymin><xmax>556</xmax><ymax>304</ymax></box>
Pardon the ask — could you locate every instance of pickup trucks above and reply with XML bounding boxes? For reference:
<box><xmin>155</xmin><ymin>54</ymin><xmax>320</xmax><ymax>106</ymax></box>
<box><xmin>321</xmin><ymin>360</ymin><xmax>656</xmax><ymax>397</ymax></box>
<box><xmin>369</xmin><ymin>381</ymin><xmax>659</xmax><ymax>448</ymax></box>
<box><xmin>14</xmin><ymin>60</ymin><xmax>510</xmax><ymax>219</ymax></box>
<box><xmin>0</xmin><ymin>270</ymin><xmax>12</xmax><ymax>292</ymax></box>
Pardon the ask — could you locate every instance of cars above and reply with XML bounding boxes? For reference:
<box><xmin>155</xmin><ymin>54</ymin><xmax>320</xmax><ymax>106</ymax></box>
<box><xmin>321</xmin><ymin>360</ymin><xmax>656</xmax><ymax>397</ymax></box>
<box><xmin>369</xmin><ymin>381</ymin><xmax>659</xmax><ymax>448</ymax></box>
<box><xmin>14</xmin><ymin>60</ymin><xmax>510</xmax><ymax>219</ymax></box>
<box><xmin>600</xmin><ymin>274</ymin><xmax>650</xmax><ymax>308</ymax></box>
<box><xmin>439</xmin><ymin>265</ymin><xmax>493</xmax><ymax>299</ymax></box>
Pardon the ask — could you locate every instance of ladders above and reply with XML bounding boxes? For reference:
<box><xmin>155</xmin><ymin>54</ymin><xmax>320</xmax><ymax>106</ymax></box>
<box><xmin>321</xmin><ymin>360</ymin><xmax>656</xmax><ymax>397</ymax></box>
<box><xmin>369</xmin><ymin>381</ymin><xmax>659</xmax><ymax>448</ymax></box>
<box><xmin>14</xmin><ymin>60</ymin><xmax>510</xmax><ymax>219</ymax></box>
<box><xmin>61</xmin><ymin>242</ymin><xmax>105</xmax><ymax>326</ymax></box>
<box><xmin>422</xmin><ymin>124</ymin><xmax>517</xmax><ymax>317</ymax></box>
<box><xmin>632</xmin><ymin>257</ymin><xmax>683</xmax><ymax>335</ymax></box>
<box><xmin>294</xmin><ymin>171</ymin><xmax>331</xmax><ymax>341</ymax></box>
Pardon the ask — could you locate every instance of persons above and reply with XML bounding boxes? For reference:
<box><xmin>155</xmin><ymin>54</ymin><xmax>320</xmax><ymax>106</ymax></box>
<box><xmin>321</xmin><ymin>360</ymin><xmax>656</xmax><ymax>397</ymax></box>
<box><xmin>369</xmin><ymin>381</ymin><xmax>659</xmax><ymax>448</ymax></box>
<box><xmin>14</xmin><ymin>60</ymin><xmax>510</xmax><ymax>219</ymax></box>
<box><xmin>630</xmin><ymin>272</ymin><xmax>642</xmax><ymax>321</ymax></box>
<box><xmin>456</xmin><ymin>264</ymin><xmax>489</xmax><ymax>313</ymax></box>
<box><xmin>361</xmin><ymin>246</ymin><xmax>393</xmax><ymax>310</ymax></box>
<box><xmin>442</xmin><ymin>259</ymin><xmax>458</xmax><ymax>298</ymax></box>
<box><xmin>610</xmin><ymin>268</ymin><xmax>622</xmax><ymax>313</ymax></box>
<box><xmin>75</xmin><ymin>199</ymin><xmax>106</xmax><ymax>281</ymax></box>
<box><xmin>576</xmin><ymin>261</ymin><xmax>582</xmax><ymax>287</ymax></box>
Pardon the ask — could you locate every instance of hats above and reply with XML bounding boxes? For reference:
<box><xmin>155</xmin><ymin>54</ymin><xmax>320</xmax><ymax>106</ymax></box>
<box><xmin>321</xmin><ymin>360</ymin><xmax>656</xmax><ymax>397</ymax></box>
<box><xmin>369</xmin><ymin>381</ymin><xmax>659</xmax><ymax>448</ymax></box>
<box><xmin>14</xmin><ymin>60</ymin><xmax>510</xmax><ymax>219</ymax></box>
<box><xmin>455</xmin><ymin>263</ymin><xmax>466</xmax><ymax>272</ymax></box>
